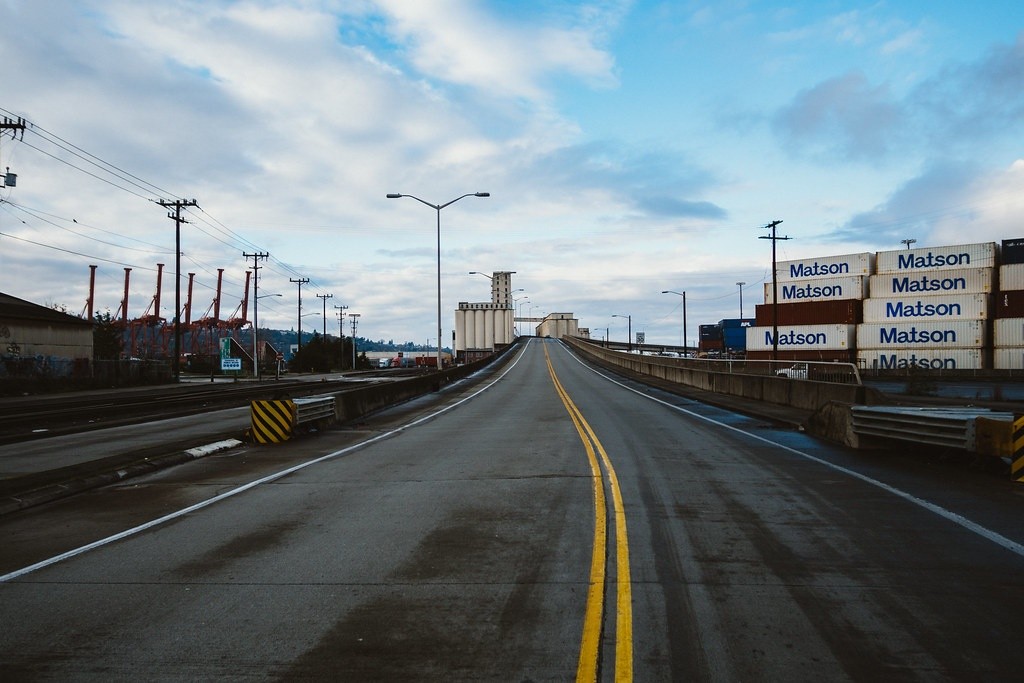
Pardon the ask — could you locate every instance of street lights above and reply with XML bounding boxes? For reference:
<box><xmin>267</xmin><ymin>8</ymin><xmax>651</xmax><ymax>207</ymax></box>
<box><xmin>469</xmin><ymin>272</ymin><xmax>516</xmax><ymax>352</ymax></box>
<box><xmin>662</xmin><ymin>291</ymin><xmax>687</xmax><ymax>357</ymax></box>
<box><xmin>736</xmin><ymin>283</ymin><xmax>745</xmax><ymax>319</ymax></box>
<box><xmin>387</xmin><ymin>192</ymin><xmax>490</xmax><ymax>370</ymax></box>
<box><xmin>612</xmin><ymin>315</ymin><xmax>631</xmax><ymax>351</ymax></box>
<box><xmin>492</xmin><ymin>289</ymin><xmax>539</xmax><ymax>336</ymax></box>
<box><xmin>299</xmin><ymin>313</ymin><xmax>320</xmax><ymax>350</ymax></box>
<box><xmin>254</xmin><ymin>294</ymin><xmax>282</xmax><ymax>378</ymax></box>
<box><xmin>595</xmin><ymin>328</ymin><xmax>609</xmax><ymax>348</ymax></box>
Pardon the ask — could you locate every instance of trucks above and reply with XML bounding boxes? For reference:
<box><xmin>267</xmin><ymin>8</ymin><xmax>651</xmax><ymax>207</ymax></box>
<box><xmin>777</xmin><ymin>358</ymin><xmax>866</xmax><ymax>383</ymax></box>
